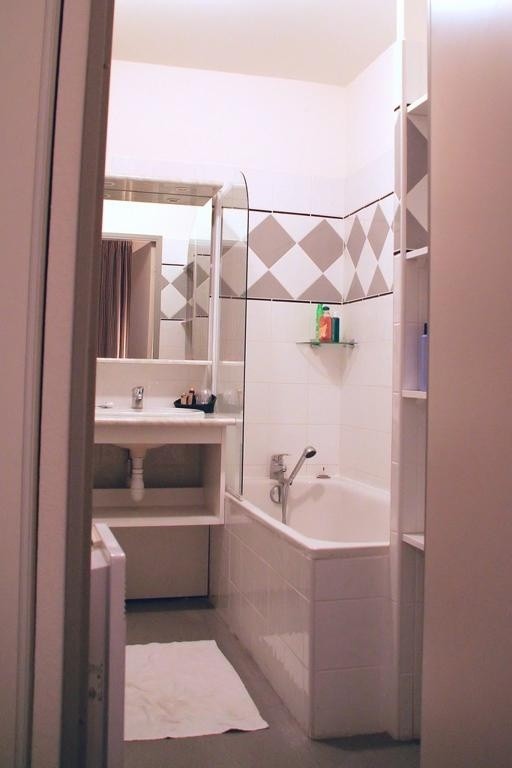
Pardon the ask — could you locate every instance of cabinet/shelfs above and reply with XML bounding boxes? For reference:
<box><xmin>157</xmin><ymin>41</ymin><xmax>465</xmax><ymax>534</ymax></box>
<box><xmin>391</xmin><ymin>103</ymin><xmax>430</xmax><ymax>551</ymax></box>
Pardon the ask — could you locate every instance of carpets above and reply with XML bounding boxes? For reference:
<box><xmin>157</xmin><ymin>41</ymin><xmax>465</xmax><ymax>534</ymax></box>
<box><xmin>122</xmin><ymin>642</ymin><xmax>267</xmax><ymax>740</ymax></box>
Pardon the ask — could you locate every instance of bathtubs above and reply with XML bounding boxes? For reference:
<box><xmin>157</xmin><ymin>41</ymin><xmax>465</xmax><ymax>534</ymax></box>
<box><xmin>208</xmin><ymin>472</ymin><xmax>392</xmax><ymax>740</ymax></box>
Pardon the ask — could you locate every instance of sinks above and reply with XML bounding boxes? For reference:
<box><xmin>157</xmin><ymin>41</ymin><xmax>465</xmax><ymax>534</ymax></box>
<box><xmin>95</xmin><ymin>407</ymin><xmax>205</xmax><ymax>418</ymax></box>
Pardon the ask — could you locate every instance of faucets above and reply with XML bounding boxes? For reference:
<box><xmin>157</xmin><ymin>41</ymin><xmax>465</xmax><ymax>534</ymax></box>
<box><xmin>269</xmin><ymin>452</ymin><xmax>291</xmax><ymax>479</ymax></box>
<box><xmin>131</xmin><ymin>385</ymin><xmax>144</xmax><ymax>410</ymax></box>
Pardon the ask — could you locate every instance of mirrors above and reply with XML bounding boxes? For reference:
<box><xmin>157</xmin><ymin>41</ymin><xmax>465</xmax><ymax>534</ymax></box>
<box><xmin>97</xmin><ymin>184</ymin><xmax>214</xmax><ymax>361</ymax></box>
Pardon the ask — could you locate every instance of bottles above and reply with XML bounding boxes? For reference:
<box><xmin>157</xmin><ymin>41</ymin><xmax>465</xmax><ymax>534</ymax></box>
<box><xmin>418</xmin><ymin>321</ymin><xmax>428</xmax><ymax>392</ymax></box>
<box><xmin>315</xmin><ymin>302</ymin><xmax>340</xmax><ymax>342</ymax></box>
